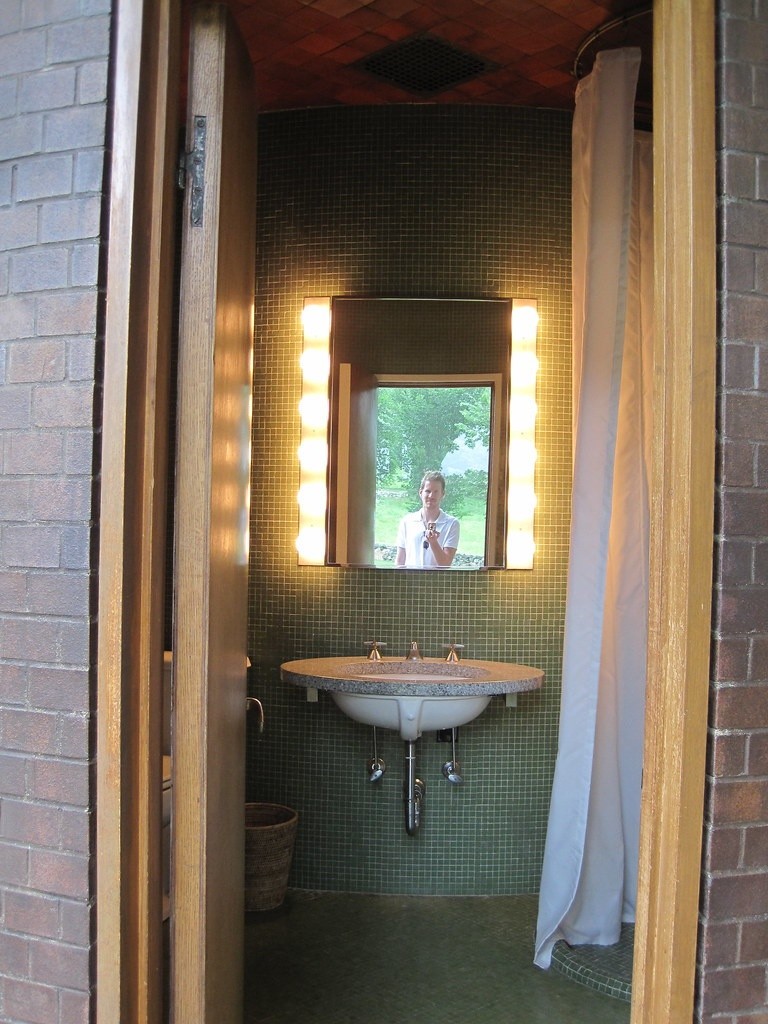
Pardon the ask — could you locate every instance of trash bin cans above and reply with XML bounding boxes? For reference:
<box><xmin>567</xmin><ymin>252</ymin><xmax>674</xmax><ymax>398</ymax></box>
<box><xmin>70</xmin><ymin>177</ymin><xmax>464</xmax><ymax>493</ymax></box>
<box><xmin>244</xmin><ymin>802</ymin><xmax>299</xmax><ymax>912</ymax></box>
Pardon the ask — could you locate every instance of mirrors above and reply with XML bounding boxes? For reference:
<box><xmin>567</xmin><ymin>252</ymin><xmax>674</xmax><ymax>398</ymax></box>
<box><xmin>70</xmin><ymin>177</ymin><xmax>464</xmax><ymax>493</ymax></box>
<box><xmin>327</xmin><ymin>296</ymin><xmax>512</xmax><ymax>570</ymax></box>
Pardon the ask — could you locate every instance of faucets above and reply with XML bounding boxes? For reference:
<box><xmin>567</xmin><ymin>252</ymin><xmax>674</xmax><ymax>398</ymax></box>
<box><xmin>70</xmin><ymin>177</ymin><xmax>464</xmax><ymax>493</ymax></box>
<box><xmin>406</xmin><ymin>640</ymin><xmax>423</xmax><ymax>662</ymax></box>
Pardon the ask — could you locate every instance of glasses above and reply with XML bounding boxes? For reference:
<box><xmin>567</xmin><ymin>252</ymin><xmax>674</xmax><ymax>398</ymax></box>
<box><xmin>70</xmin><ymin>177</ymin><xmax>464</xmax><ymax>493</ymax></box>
<box><xmin>423</xmin><ymin>530</ymin><xmax>429</xmax><ymax>550</ymax></box>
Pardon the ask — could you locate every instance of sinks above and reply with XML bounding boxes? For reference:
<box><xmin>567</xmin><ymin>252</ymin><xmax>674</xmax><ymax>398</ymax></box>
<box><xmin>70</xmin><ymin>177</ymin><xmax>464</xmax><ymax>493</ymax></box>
<box><xmin>278</xmin><ymin>656</ymin><xmax>546</xmax><ymax>741</ymax></box>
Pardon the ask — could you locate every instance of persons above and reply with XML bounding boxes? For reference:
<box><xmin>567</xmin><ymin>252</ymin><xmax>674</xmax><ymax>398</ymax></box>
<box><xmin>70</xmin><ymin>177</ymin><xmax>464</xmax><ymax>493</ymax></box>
<box><xmin>395</xmin><ymin>470</ymin><xmax>460</xmax><ymax>568</ymax></box>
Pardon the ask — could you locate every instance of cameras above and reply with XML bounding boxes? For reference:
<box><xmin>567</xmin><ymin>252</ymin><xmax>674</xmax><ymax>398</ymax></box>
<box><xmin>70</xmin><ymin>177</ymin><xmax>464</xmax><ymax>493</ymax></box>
<box><xmin>428</xmin><ymin>522</ymin><xmax>437</xmax><ymax>533</ymax></box>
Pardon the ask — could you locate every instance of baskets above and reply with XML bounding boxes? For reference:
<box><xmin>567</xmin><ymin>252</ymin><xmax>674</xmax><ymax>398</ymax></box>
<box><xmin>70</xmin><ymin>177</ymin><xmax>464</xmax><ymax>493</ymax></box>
<box><xmin>243</xmin><ymin>803</ymin><xmax>299</xmax><ymax>912</ymax></box>
